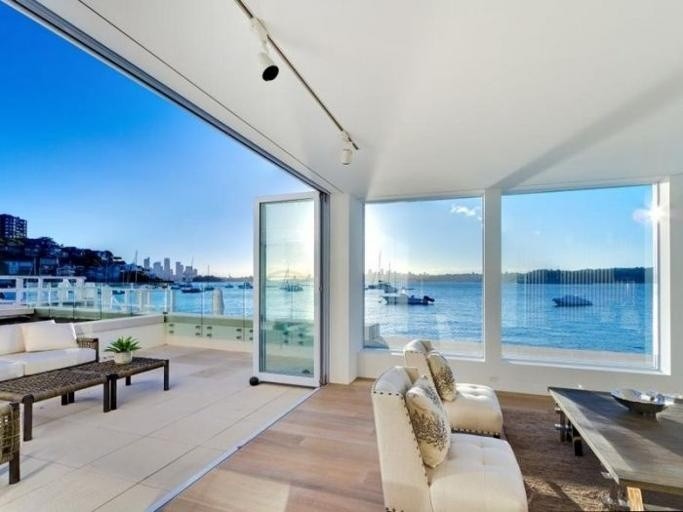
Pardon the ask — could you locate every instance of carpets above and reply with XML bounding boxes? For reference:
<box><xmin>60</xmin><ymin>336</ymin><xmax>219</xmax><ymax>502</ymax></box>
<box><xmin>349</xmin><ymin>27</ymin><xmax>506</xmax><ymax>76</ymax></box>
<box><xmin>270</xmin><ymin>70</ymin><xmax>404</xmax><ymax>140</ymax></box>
<box><xmin>502</xmin><ymin>407</ymin><xmax>683</xmax><ymax>512</ymax></box>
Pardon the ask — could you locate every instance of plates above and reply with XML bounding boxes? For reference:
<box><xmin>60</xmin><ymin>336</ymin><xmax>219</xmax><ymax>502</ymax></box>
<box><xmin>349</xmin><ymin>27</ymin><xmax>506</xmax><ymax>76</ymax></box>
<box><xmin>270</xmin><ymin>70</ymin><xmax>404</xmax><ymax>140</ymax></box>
<box><xmin>610</xmin><ymin>388</ymin><xmax>678</xmax><ymax>423</ymax></box>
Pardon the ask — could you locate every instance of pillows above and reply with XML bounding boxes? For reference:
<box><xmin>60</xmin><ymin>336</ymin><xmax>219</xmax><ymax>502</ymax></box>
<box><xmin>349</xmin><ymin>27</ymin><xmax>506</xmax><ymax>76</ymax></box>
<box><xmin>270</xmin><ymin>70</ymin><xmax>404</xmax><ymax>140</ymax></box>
<box><xmin>22</xmin><ymin>323</ymin><xmax>80</xmax><ymax>352</ymax></box>
<box><xmin>405</xmin><ymin>374</ymin><xmax>451</xmax><ymax>469</ymax></box>
<box><xmin>428</xmin><ymin>345</ymin><xmax>456</xmax><ymax>401</ymax></box>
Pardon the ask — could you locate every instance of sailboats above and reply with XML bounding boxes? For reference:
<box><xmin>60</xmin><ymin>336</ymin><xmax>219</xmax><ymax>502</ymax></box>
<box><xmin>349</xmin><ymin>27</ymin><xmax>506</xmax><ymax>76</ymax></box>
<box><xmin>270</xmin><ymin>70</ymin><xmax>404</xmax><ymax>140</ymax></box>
<box><xmin>0</xmin><ymin>250</ymin><xmax>214</xmax><ymax>310</ymax></box>
<box><xmin>279</xmin><ymin>269</ymin><xmax>303</xmax><ymax>291</ymax></box>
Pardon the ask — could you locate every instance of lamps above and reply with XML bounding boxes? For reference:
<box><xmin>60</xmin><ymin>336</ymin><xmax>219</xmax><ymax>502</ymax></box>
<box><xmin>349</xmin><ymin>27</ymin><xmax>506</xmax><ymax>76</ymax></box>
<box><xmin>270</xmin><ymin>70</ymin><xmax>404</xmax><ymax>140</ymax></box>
<box><xmin>342</xmin><ymin>148</ymin><xmax>352</xmax><ymax>165</ymax></box>
<box><xmin>257</xmin><ymin>51</ymin><xmax>279</xmax><ymax>81</ymax></box>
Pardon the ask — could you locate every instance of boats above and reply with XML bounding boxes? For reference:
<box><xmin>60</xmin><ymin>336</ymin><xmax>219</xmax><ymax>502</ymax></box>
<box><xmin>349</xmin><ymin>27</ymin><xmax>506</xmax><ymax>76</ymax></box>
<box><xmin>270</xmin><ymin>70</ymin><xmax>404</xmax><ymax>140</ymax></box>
<box><xmin>225</xmin><ymin>285</ymin><xmax>233</xmax><ymax>288</ymax></box>
<box><xmin>238</xmin><ymin>282</ymin><xmax>252</xmax><ymax>288</ymax></box>
<box><xmin>369</xmin><ymin>281</ymin><xmax>434</xmax><ymax>304</ymax></box>
<box><xmin>552</xmin><ymin>295</ymin><xmax>592</xmax><ymax>305</ymax></box>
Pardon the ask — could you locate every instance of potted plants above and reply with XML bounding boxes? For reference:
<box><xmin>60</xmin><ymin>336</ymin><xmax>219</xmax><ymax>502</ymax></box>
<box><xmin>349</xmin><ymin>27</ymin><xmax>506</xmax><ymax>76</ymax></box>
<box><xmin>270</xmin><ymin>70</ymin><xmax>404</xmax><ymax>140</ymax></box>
<box><xmin>105</xmin><ymin>336</ymin><xmax>143</xmax><ymax>365</ymax></box>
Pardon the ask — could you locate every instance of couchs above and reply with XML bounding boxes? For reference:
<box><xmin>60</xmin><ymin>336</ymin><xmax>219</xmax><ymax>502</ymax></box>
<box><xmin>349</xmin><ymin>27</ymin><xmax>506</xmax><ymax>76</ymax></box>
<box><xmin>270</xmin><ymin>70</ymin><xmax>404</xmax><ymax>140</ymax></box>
<box><xmin>0</xmin><ymin>319</ymin><xmax>99</xmax><ymax>383</ymax></box>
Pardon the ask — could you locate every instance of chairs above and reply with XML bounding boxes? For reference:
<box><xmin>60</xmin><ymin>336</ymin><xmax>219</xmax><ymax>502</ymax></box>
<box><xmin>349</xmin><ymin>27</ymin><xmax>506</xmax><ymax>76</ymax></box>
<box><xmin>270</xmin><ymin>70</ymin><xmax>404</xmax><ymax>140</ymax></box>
<box><xmin>401</xmin><ymin>339</ymin><xmax>505</xmax><ymax>439</ymax></box>
<box><xmin>0</xmin><ymin>400</ymin><xmax>20</xmax><ymax>483</ymax></box>
<box><xmin>371</xmin><ymin>364</ymin><xmax>529</xmax><ymax>512</ymax></box>
<box><xmin>0</xmin><ymin>367</ymin><xmax>110</xmax><ymax>441</ymax></box>
